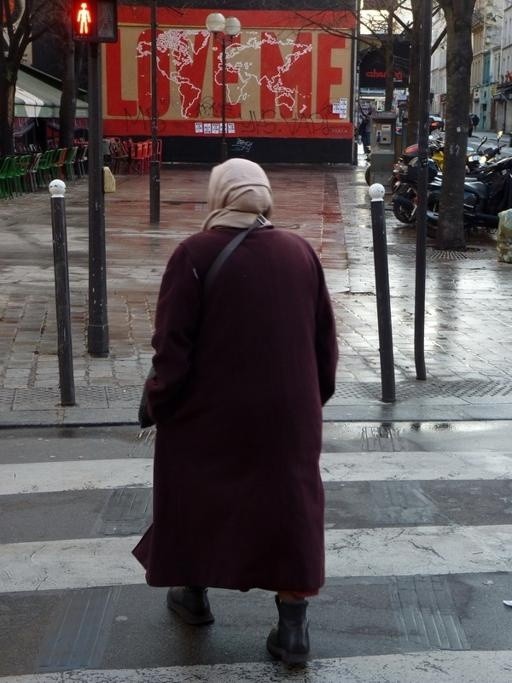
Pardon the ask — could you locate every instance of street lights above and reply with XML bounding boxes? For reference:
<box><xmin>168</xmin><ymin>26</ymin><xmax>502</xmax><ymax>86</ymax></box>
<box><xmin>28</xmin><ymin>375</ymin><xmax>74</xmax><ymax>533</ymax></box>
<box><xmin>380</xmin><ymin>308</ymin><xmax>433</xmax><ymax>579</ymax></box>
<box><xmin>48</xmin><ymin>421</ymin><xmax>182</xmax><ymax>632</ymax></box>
<box><xmin>206</xmin><ymin>12</ymin><xmax>241</xmax><ymax>164</ymax></box>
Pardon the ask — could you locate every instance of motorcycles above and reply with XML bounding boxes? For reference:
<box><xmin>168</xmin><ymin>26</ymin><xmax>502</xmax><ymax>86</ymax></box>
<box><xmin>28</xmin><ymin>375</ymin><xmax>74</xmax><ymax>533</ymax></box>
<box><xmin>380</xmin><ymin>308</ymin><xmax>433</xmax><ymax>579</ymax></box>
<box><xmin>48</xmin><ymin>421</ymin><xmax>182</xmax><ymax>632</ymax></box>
<box><xmin>392</xmin><ymin>131</ymin><xmax>512</xmax><ymax>238</ymax></box>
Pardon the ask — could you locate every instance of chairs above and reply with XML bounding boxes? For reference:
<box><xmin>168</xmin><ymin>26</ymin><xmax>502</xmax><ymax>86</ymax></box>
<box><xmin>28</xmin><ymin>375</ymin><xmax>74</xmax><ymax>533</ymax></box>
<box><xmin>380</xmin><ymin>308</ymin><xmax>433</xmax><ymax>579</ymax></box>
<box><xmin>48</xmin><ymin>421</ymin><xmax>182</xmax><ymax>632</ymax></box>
<box><xmin>0</xmin><ymin>137</ymin><xmax>162</xmax><ymax>200</ymax></box>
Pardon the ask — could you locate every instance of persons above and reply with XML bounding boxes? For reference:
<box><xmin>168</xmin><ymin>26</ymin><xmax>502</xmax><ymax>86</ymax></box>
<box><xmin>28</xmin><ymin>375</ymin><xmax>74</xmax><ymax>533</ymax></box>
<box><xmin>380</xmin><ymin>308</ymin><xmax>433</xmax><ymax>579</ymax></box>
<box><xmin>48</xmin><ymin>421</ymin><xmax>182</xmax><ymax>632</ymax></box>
<box><xmin>472</xmin><ymin>114</ymin><xmax>480</xmax><ymax>132</ymax></box>
<box><xmin>130</xmin><ymin>158</ymin><xmax>338</xmax><ymax>667</ymax></box>
<box><xmin>358</xmin><ymin>106</ymin><xmax>377</xmax><ymax>163</ymax></box>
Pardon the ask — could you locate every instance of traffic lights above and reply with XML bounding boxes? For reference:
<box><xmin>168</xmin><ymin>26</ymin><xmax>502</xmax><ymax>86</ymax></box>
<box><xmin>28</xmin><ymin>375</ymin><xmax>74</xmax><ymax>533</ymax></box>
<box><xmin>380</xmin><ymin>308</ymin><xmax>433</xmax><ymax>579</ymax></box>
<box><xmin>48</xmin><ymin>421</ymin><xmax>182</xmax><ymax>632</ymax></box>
<box><xmin>70</xmin><ymin>0</ymin><xmax>118</xmax><ymax>45</ymax></box>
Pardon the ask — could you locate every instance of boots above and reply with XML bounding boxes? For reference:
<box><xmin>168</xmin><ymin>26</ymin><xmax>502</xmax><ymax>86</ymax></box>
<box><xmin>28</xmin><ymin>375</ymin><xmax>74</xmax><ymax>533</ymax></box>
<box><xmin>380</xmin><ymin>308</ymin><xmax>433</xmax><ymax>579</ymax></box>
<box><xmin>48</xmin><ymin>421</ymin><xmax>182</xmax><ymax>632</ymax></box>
<box><xmin>167</xmin><ymin>586</ymin><xmax>215</xmax><ymax>625</ymax></box>
<box><xmin>267</xmin><ymin>592</ymin><xmax>309</xmax><ymax>664</ymax></box>
